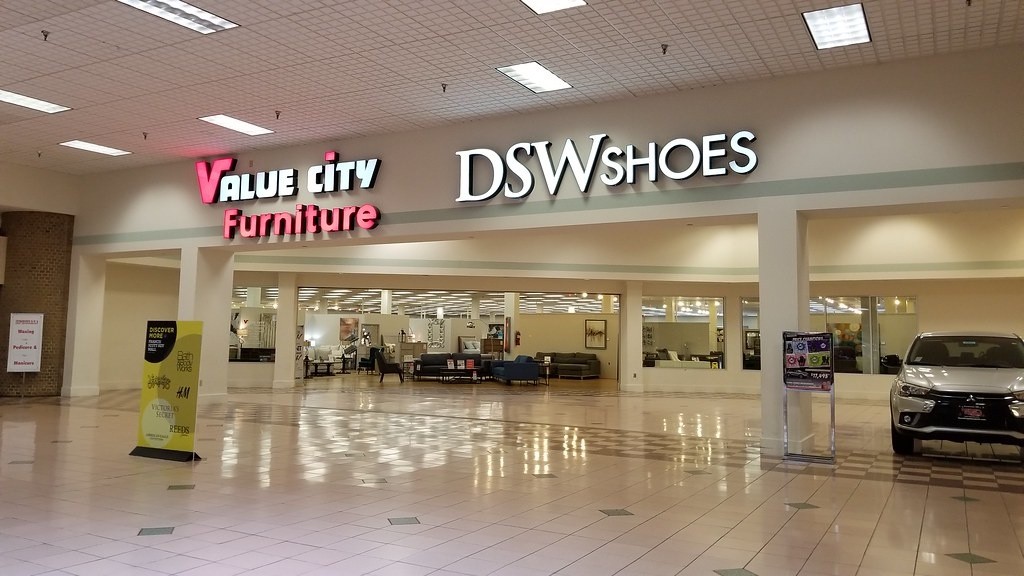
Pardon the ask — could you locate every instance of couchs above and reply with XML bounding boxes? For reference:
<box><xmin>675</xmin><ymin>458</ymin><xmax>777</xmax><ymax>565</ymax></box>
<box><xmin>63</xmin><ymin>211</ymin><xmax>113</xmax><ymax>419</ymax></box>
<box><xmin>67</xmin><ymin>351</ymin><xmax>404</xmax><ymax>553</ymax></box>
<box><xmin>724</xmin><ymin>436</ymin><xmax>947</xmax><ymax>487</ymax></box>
<box><xmin>490</xmin><ymin>355</ymin><xmax>541</xmax><ymax>385</ymax></box>
<box><xmin>413</xmin><ymin>352</ymin><xmax>491</xmax><ymax>381</ymax></box>
<box><xmin>533</xmin><ymin>352</ymin><xmax>601</xmax><ymax>380</ymax></box>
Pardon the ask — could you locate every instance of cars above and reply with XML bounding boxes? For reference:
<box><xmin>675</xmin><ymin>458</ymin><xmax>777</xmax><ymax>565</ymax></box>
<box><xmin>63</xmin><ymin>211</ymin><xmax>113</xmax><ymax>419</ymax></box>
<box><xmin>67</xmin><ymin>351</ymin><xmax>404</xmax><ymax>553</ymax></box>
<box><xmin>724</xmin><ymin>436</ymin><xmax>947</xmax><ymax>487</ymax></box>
<box><xmin>879</xmin><ymin>330</ymin><xmax>1024</xmax><ymax>457</ymax></box>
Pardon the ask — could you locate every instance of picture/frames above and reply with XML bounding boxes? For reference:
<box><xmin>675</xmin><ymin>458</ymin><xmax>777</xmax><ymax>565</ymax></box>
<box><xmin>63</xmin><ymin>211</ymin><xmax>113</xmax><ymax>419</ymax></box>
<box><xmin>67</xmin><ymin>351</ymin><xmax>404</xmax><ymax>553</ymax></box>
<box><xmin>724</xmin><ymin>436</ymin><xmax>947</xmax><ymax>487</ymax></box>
<box><xmin>585</xmin><ymin>319</ymin><xmax>607</xmax><ymax>349</ymax></box>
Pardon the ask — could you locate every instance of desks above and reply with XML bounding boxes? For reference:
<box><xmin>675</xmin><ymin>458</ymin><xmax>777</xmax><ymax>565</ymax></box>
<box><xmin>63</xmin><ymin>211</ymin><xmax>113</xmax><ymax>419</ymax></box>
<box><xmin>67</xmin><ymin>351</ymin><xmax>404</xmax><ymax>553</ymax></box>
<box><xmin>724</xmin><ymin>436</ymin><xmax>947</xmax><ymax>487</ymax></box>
<box><xmin>311</xmin><ymin>362</ymin><xmax>337</xmax><ymax>375</ymax></box>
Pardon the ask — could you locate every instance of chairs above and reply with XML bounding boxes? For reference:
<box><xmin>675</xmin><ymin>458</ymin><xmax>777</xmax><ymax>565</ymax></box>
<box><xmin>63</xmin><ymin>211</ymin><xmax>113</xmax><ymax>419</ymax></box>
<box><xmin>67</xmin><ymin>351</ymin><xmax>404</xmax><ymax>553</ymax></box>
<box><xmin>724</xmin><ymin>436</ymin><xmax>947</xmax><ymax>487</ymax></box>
<box><xmin>357</xmin><ymin>356</ymin><xmax>374</xmax><ymax>376</ymax></box>
<box><xmin>986</xmin><ymin>347</ymin><xmax>1005</xmax><ymax>363</ymax></box>
<box><xmin>923</xmin><ymin>340</ymin><xmax>948</xmax><ymax>361</ymax></box>
<box><xmin>376</xmin><ymin>350</ymin><xmax>405</xmax><ymax>383</ymax></box>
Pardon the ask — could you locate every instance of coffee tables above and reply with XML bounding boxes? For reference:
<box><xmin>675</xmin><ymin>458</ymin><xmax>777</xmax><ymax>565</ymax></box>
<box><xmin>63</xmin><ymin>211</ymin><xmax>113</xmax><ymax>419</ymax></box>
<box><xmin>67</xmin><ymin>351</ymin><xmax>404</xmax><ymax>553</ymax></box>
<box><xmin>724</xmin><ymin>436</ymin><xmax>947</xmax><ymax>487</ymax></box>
<box><xmin>440</xmin><ymin>367</ymin><xmax>479</xmax><ymax>384</ymax></box>
